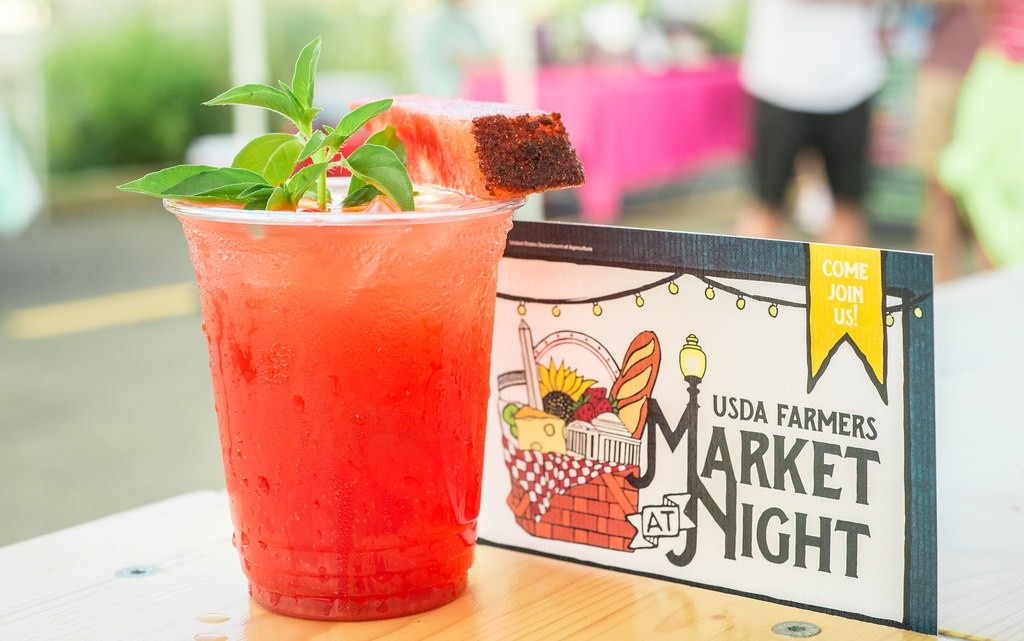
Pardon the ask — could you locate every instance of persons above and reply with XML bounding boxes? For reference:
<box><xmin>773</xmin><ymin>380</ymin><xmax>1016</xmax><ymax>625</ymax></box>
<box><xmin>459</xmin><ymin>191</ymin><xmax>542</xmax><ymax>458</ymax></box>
<box><xmin>905</xmin><ymin>1</ymin><xmax>1024</xmax><ymax>283</ymax></box>
<box><xmin>738</xmin><ymin>0</ymin><xmax>891</xmax><ymax>247</ymax></box>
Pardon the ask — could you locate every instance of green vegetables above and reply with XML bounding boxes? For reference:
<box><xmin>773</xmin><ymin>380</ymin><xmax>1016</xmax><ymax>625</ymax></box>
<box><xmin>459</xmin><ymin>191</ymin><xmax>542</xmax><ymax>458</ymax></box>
<box><xmin>112</xmin><ymin>36</ymin><xmax>420</xmax><ymax>215</ymax></box>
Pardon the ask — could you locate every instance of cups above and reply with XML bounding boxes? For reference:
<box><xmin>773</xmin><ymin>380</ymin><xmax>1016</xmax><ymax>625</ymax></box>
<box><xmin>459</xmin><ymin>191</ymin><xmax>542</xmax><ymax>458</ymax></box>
<box><xmin>170</xmin><ymin>189</ymin><xmax>526</xmax><ymax>621</ymax></box>
